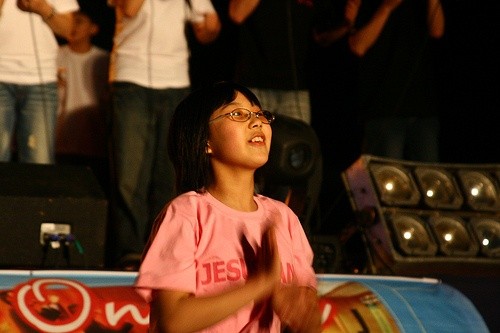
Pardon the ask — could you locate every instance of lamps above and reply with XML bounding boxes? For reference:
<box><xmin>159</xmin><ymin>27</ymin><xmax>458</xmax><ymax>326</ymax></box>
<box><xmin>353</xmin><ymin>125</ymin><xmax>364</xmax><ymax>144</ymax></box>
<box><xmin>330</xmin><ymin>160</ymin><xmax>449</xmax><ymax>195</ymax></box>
<box><xmin>341</xmin><ymin>153</ymin><xmax>500</xmax><ymax>278</ymax></box>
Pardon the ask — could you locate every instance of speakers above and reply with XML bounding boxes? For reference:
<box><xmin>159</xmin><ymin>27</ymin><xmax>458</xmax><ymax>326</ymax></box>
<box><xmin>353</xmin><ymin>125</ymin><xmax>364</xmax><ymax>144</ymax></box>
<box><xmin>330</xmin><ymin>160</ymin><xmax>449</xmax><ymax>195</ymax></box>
<box><xmin>0</xmin><ymin>160</ymin><xmax>108</xmax><ymax>270</ymax></box>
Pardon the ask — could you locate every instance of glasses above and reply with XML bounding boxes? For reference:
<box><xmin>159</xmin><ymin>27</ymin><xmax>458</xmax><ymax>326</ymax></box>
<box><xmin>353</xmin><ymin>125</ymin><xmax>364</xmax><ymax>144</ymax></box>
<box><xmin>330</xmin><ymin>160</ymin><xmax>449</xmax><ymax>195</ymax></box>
<box><xmin>208</xmin><ymin>108</ymin><xmax>276</xmax><ymax>124</ymax></box>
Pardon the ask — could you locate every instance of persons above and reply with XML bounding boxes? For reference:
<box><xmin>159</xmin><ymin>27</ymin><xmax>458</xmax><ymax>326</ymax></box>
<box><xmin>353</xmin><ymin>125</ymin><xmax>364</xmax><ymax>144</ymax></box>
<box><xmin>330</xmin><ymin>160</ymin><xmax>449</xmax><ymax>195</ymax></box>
<box><xmin>0</xmin><ymin>0</ymin><xmax>461</xmax><ymax>237</ymax></box>
<box><xmin>133</xmin><ymin>79</ymin><xmax>324</xmax><ymax>333</ymax></box>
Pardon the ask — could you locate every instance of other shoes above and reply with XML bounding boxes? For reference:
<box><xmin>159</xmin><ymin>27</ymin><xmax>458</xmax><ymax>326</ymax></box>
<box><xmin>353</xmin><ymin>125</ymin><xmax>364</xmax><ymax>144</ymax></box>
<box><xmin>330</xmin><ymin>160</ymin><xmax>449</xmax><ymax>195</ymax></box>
<box><xmin>120</xmin><ymin>251</ymin><xmax>137</xmax><ymax>270</ymax></box>
